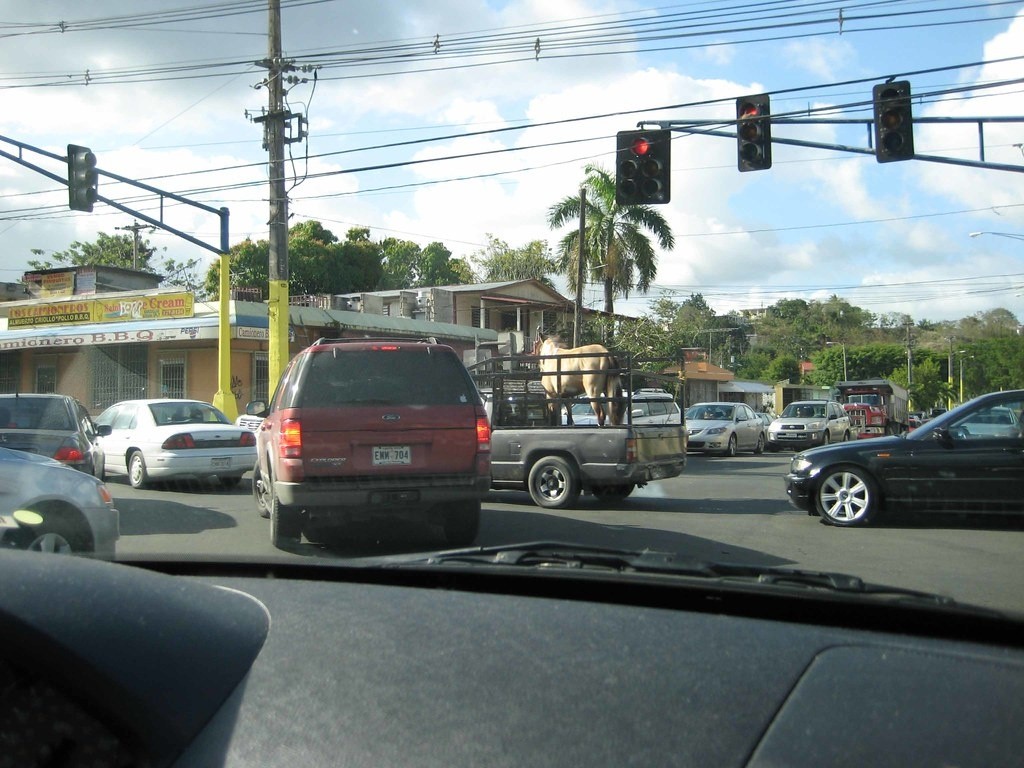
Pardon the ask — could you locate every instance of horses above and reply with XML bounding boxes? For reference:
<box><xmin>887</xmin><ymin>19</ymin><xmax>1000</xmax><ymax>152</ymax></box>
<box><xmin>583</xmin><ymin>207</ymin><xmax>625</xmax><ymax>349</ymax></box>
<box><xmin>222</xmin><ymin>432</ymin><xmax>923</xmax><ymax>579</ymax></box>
<box><xmin>535</xmin><ymin>332</ymin><xmax>626</xmax><ymax>427</ymax></box>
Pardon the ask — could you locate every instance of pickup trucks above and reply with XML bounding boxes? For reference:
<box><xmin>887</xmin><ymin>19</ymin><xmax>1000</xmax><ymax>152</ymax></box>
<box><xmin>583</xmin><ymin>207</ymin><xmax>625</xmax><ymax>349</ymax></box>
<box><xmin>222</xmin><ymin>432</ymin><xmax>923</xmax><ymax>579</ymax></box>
<box><xmin>466</xmin><ymin>350</ymin><xmax>688</xmax><ymax>508</ymax></box>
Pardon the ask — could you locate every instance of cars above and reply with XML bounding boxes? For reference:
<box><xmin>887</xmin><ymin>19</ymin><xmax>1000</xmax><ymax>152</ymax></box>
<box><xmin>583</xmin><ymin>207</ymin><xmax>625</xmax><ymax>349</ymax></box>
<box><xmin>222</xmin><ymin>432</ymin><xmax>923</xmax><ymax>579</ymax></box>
<box><xmin>94</xmin><ymin>399</ymin><xmax>264</xmax><ymax>490</ymax></box>
<box><xmin>1</xmin><ymin>393</ymin><xmax>120</xmax><ymax>559</ymax></box>
<box><xmin>685</xmin><ymin>400</ymin><xmax>859</xmax><ymax>456</ymax></box>
<box><xmin>784</xmin><ymin>388</ymin><xmax>1024</xmax><ymax>527</ymax></box>
<box><xmin>910</xmin><ymin>402</ymin><xmax>1021</xmax><ymax>439</ymax></box>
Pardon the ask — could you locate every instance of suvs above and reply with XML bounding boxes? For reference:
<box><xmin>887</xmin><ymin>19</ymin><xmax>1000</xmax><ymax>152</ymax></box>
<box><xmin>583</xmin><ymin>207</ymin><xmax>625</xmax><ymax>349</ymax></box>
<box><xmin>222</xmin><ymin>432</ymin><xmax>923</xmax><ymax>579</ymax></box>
<box><xmin>252</xmin><ymin>337</ymin><xmax>492</xmax><ymax>551</ymax></box>
<box><xmin>562</xmin><ymin>387</ymin><xmax>682</xmax><ymax>425</ymax></box>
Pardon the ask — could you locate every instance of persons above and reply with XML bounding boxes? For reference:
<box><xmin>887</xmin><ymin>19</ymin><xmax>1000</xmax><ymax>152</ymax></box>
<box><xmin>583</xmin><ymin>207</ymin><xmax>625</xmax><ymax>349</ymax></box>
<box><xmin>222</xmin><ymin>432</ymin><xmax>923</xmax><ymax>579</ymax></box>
<box><xmin>796</xmin><ymin>406</ymin><xmax>806</xmax><ymax>417</ymax></box>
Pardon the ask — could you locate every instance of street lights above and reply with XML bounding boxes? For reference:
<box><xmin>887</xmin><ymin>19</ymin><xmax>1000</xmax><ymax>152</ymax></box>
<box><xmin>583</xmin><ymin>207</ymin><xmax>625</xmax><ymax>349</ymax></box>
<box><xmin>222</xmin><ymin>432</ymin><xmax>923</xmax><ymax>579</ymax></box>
<box><xmin>960</xmin><ymin>356</ymin><xmax>975</xmax><ymax>404</ymax></box>
<box><xmin>948</xmin><ymin>350</ymin><xmax>966</xmax><ymax>411</ymax></box>
<box><xmin>826</xmin><ymin>341</ymin><xmax>846</xmax><ymax>382</ymax></box>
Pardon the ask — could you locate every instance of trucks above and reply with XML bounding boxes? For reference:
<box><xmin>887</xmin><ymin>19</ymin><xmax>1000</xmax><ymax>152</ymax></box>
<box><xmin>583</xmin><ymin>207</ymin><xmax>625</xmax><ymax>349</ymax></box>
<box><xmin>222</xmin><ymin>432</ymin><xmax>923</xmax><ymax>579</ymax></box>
<box><xmin>834</xmin><ymin>380</ymin><xmax>909</xmax><ymax>440</ymax></box>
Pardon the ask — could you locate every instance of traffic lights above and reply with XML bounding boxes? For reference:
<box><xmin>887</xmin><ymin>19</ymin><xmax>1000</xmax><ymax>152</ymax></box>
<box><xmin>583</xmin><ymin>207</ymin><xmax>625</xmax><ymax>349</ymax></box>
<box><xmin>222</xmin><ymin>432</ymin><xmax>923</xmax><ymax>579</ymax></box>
<box><xmin>615</xmin><ymin>129</ymin><xmax>671</xmax><ymax>205</ymax></box>
<box><xmin>736</xmin><ymin>95</ymin><xmax>772</xmax><ymax>172</ymax></box>
<box><xmin>872</xmin><ymin>80</ymin><xmax>915</xmax><ymax>164</ymax></box>
<box><xmin>67</xmin><ymin>144</ymin><xmax>98</xmax><ymax>213</ymax></box>
